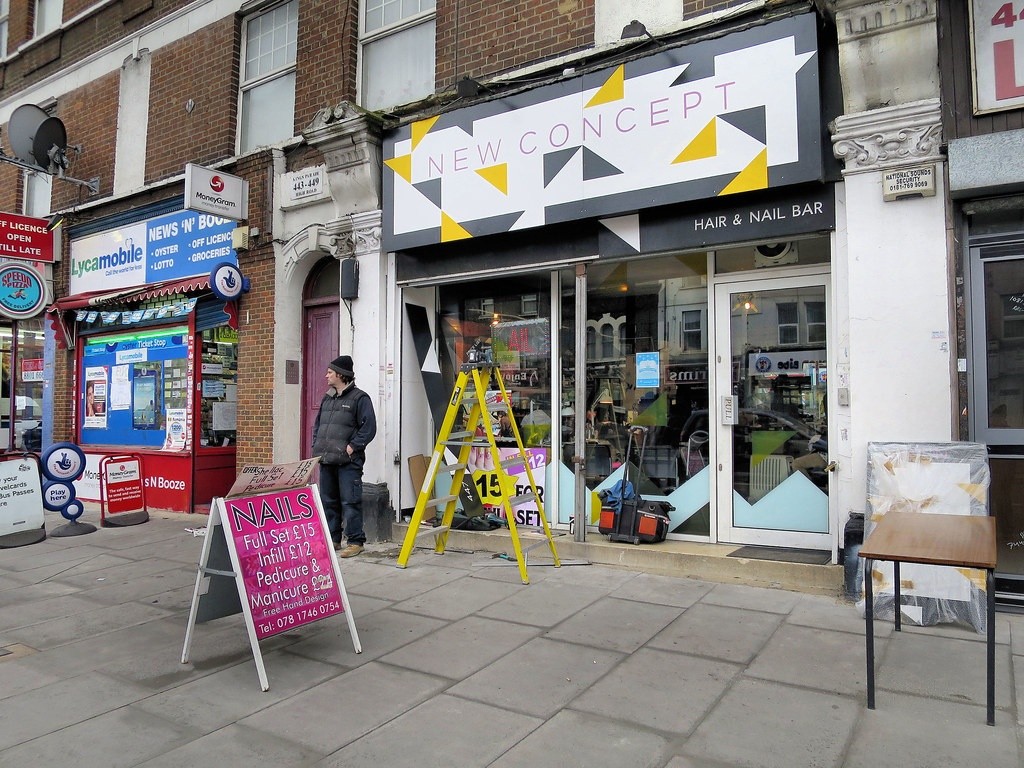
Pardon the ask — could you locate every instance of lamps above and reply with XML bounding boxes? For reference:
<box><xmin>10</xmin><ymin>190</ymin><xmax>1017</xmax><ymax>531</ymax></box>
<box><xmin>622</xmin><ymin>20</ymin><xmax>654</xmax><ymax>41</ymax></box>
<box><xmin>459</xmin><ymin>76</ymin><xmax>494</xmax><ymax>96</ymax></box>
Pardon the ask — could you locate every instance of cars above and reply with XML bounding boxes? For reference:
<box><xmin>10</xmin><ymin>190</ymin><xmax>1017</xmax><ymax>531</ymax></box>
<box><xmin>678</xmin><ymin>406</ymin><xmax>830</xmax><ymax>492</ymax></box>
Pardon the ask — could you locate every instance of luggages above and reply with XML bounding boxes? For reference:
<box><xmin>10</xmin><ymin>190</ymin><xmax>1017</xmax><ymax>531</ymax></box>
<box><xmin>600</xmin><ymin>425</ymin><xmax>675</xmax><ymax>545</ymax></box>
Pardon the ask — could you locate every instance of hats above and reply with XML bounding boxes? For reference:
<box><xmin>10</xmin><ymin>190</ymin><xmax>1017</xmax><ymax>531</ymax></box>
<box><xmin>328</xmin><ymin>355</ymin><xmax>355</xmax><ymax>377</ymax></box>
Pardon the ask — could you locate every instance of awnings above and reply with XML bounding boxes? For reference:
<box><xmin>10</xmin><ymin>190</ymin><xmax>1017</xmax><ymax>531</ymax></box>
<box><xmin>45</xmin><ymin>273</ymin><xmax>239</xmax><ymax>353</ymax></box>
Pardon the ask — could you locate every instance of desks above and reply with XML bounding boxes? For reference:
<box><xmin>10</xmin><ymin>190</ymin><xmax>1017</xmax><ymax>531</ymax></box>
<box><xmin>859</xmin><ymin>512</ymin><xmax>998</xmax><ymax>726</ymax></box>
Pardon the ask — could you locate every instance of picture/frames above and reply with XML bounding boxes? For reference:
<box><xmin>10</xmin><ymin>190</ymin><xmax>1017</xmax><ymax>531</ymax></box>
<box><xmin>864</xmin><ymin>441</ymin><xmax>990</xmax><ymax>627</ymax></box>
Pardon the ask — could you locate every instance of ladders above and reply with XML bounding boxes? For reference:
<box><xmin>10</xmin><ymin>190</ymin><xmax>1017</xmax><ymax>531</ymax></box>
<box><xmin>396</xmin><ymin>362</ymin><xmax>561</xmax><ymax>585</ymax></box>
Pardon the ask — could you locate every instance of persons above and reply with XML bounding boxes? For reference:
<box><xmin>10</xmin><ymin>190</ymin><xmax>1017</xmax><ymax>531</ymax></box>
<box><xmin>313</xmin><ymin>355</ymin><xmax>378</xmax><ymax>559</ymax></box>
<box><xmin>85</xmin><ymin>382</ymin><xmax>98</xmax><ymax>417</ymax></box>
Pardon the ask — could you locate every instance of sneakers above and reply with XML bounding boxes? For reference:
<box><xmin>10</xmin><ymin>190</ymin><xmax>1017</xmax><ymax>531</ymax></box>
<box><xmin>340</xmin><ymin>544</ymin><xmax>365</xmax><ymax>558</ymax></box>
<box><xmin>332</xmin><ymin>542</ymin><xmax>341</xmax><ymax>551</ymax></box>
